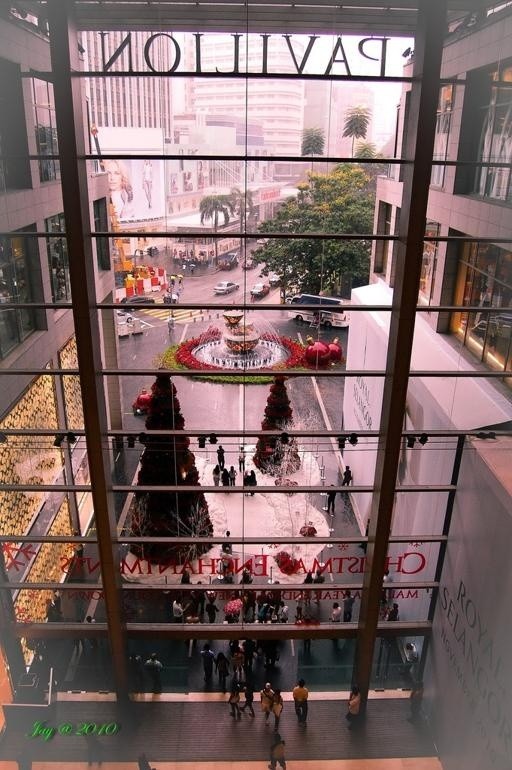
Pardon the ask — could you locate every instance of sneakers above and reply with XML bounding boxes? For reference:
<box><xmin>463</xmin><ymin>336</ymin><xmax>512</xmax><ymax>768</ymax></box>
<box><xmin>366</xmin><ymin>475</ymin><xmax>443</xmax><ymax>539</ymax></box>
<box><xmin>230</xmin><ymin>705</ymin><xmax>278</xmax><ymax>730</ymax></box>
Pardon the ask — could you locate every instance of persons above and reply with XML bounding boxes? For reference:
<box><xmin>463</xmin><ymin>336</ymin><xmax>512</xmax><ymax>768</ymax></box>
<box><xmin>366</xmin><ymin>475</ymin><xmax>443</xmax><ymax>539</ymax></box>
<box><xmin>160</xmin><ymin>262</ymin><xmax>198</xmax><ymax>337</ymax></box>
<box><xmin>243</xmin><ymin>470</ymin><xmax>250</xmax><ymax>497</ymax></box>
<box><xmin>228</xmin><ymin>465</ymin><xmax>237</xmax><ymax>486</ymax></box>
<box><xmin>141</xmin><ymin>160</ymin><xmax>157</xmax><ymax>211</ymax></box>
<box><xmin>216</xmin><ymin>445</ymin><xmax>225</xmax><ymax>471</ymax></box>
<box><xmin>249</xmin><ymin>470</ymin><xmax>257</xmax><ymax>496</ymax></box>
<box><xmin>238</xmin><ymin>446</ymin><xmax>246</xmax><ymax>473</ymax></box>
<box><xmin>212</xmin><ymin>464</ymin><xmax>221</xmax><ymax>486</ymax></box>
<box><xmin>222</xmin><ymin>468</ymin><xmax>229</xmax><ymax>486</ymax></box>
<box><xmin>1</xmin><ymin>529</ymin><xmax>426</xmax><ymax>770</ymax></box>
<box><xmin>325</xmin><ymin>483</ymin><xmax>337</xmax><ymax>514</ymax></box>
<box><xmin>105</xmin><ymin>157</ymin><xmax>136</xmax><ymax>217</ymax></box>
<box><xmin>342</xmin><ymin>466</ymin><xmax>352</xmax><ymax>486</ymax></box>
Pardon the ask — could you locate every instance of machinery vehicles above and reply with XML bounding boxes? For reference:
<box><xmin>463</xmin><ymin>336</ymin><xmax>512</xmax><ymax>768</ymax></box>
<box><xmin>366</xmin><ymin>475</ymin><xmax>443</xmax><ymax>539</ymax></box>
<box><xmin>109</xmin><ymin>198</ymin><xmax>135</xmax><ymax>281</ymax></box>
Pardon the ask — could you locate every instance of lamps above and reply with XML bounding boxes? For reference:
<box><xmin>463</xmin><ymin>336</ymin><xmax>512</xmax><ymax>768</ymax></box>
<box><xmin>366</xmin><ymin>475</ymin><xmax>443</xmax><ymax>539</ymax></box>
<box><xmin>1</xmin><ymin>430</ymin><xmax>499</xmax><ymax>450</ymax></box>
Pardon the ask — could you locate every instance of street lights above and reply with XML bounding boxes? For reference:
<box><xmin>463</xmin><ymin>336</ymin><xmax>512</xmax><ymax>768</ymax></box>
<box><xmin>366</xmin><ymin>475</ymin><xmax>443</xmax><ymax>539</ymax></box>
<box><xmin>134</xmin><ymin>249</ymin><xmax>144</xmax><ymax>272</ymax></box>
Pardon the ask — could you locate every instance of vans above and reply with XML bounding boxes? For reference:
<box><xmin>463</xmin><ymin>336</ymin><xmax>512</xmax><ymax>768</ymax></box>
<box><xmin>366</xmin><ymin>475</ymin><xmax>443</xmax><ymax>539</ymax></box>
<box><xmin>285</xmin><ymin>293</ymin><xmax>350</xmax><ymax>329</ymax></box>
<box><xmin>490</xmin><ymin>315</ymin><xmax>512</xmax><ymax>327</ymax></box>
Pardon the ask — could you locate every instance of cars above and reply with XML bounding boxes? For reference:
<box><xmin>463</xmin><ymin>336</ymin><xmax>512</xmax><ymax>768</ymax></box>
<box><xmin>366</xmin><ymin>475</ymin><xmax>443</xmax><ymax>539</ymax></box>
<box><xmin>250</xmin><ymin>283</ymin><xmax>270</xmax><ymax>299</ymax></box>
<box><xmin>269</xmin><ymin>274</ymin><xmax>286</xmax><ymax>287</ymax></box>
<box><xmin>214</xmin><ymin>281</ymin><xmax>239</xmax><ymax>295</ymax></box>
<box><xmin>242</xmin><ymin>260</ymin><xmax>256</xmax><ymax>269</ymax></box>
<box><xmin>125</xmin><ymin>296</ymin><xmax>155</xmax><ymax>313</ymax></box>
